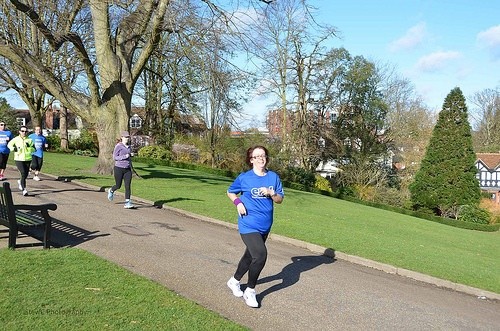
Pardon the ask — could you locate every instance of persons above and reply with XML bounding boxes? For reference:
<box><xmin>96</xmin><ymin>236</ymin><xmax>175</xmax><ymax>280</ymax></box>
<box><xmin>27</xmin><ymin>125</ymin><xmax>48</xmax><ymax>180</ymax></box>
<box><xmin>0</xmin><ymin>120</ymin><xmax>14</xmax><ymax>180</ymax></box>
<box><xmin>226</xmin><ymin>146</ymin><xmax>284</xmax><ymax>307</ymax></box>
<box><xmin>108</xmin><ymin>131</ymin><xmax>134</xmax><ymax>209</ymax></box>
<box><xmin>7</xmin><ymin>126</ymin><xmax>37</xmax><ymax>196</ymax></box>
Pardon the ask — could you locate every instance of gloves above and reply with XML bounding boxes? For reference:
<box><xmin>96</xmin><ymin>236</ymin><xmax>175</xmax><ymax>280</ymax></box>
<box><xmin>26</xmin><ymin>143</ymin><xmax>29</xmax><ymax>147</ymax></box>
<box><xmin>14</xmin><ymin>147</ymin><xmax>17</xmax><ymax>151</ymax></box>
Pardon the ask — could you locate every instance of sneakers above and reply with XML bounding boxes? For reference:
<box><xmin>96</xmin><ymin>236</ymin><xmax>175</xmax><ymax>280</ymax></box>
<box><xmin>227</xmin><ymin>276</ymin><xmax>243</xmax><ymax>297</ymax></box>
<box><xmin>23</xmin><ymin>189</ymin><xmax>28</xmax><ymax>196</ymax></box>
<box><xmin>108</xmin><ymin>188</ymin><xmax>113</xmax><ymax>201</ymax></box>
<box><xmin>18</xmin><ymin>179</ymin><xmax>23</xmax><ymax>190</ymax></box>
<box><xmin>33</xmin><ymin>176</ymin><xmax>40</xmax><ymax>181</ymax></box>
<box><xmin>124</xmin><ymin>202</ymin><xmax>134</xmax><ymax>209</ymax></box>
<box><xmin>242</xmin><ymin>289</ymin><xmax>258</xmax><ymax>307</ymax></box>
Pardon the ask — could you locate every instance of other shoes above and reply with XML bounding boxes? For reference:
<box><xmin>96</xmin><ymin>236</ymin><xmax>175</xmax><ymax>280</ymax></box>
<box><xmin>0</xmin><ymin>174</ymin><xmax>7</xmax><ymax>181</ymax></box>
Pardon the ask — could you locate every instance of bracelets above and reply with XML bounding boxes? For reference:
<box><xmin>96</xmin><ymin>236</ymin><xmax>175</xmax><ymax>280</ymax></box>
<box><xmin>234</xmin><ymin>198</ymin><xmax>242</xmax><ymax>205</ymax></box>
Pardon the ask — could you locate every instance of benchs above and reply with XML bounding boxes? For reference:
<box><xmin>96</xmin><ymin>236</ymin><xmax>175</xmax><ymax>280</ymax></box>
<box><xmin>0</xmin><ymin>181</ymin><xmax>58</xmax><ymax>251</ymax></box>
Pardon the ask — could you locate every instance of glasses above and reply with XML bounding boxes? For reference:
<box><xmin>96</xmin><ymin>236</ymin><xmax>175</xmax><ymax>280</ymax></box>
<box><xmin>251</xmin><ymin>155</ymin><xmax>267</xmax><ymax>159</ymax></box>
<box><xmin>20</xmin><ymin>130</ymin><xmax>28</xmax><ymax>132</ymax></box>
<box><xmin>0</xmin><ymin>124</ymin><xmax>6</xmax><ymax>126</ymax></box>
